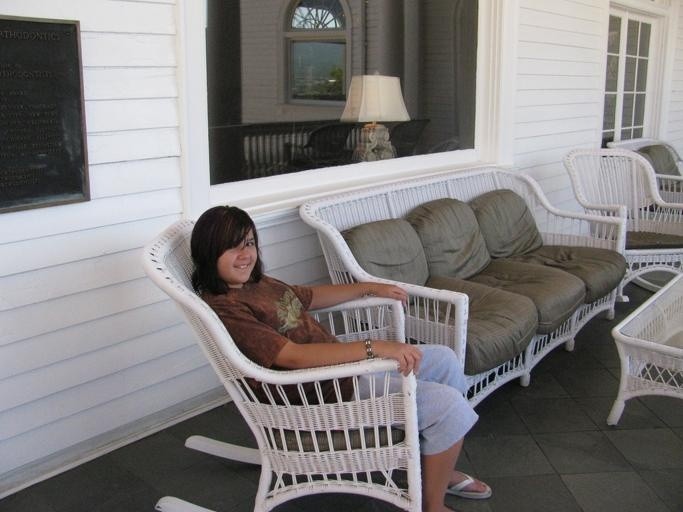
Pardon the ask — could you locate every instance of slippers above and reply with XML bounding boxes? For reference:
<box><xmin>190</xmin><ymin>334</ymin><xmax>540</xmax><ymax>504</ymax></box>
<box><xmin>446</xmin><ymin>470</ymin><xmax>492</xmax><ymax>499</ymax></box>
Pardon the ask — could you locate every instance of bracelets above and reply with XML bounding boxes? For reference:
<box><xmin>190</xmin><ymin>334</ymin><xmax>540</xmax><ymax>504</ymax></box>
<box><xmin>365</xmin><ymin>338</ymin><xmax>373</xmax><ymax>358</ymax></box>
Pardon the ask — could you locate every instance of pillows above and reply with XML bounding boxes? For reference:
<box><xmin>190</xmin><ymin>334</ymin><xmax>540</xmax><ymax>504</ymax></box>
<box><xmin>406</xmin><ymin>198</ymin><xmax>492</xmax><ymax>280</ymax></box>
<box><xmin>632</xmin><ymin>150</ymin><xmax>656</xmax><ymax>173</ymax></box>
<box><xmin>340</xmin><ymin>218</ymin><xmax>430</xmax><ymax>305</ymax></box>
<box><xmin>468</xmin><ymin>189</ymin><xmax>544</xmax><ymax>258</ymax></box>
<box><xmin>639</xmin><ymin>145</ymin><xmax>682</xmax><ymax>187</ymax></box>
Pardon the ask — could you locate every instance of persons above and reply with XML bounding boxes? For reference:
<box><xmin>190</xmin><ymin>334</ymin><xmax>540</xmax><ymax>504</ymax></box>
<box><xmin>189</xmin><ymin>205</ymin><xmax>492</xmax><ymax>512</ymax></box>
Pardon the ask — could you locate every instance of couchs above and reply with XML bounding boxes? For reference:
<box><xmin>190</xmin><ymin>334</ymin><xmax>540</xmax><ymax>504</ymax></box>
<box><xmin>606</xmin><ymin>137</ymin><xmax>683</xmax><ymax>223</ymax></box>
<box><xmin>298</xmin><ymin>164</ymin><xmax>626</xmax><ymax>410</ymax></box>
<box><xmin>282</xmin><ymin>114</ymin><xmax>431</xmax><ymax>175</ymax></box>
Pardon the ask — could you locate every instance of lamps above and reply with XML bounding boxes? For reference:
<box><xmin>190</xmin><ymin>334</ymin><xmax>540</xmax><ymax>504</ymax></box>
<box><xmin>339</xmin><ymin>71</ymin><xmax>413</xmax><ymax>164</ymax></box>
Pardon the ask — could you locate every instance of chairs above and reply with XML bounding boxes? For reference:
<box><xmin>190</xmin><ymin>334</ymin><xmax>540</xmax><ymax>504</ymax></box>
<box><xmin>138</xmin><ymin>217</ymin><xmax>470</xmax><ymax>512</ymax></box>
<box><xmin>563</xmin><ymin>147</ymin><xmax>683</xmax><ymax>303</ymax></box>
